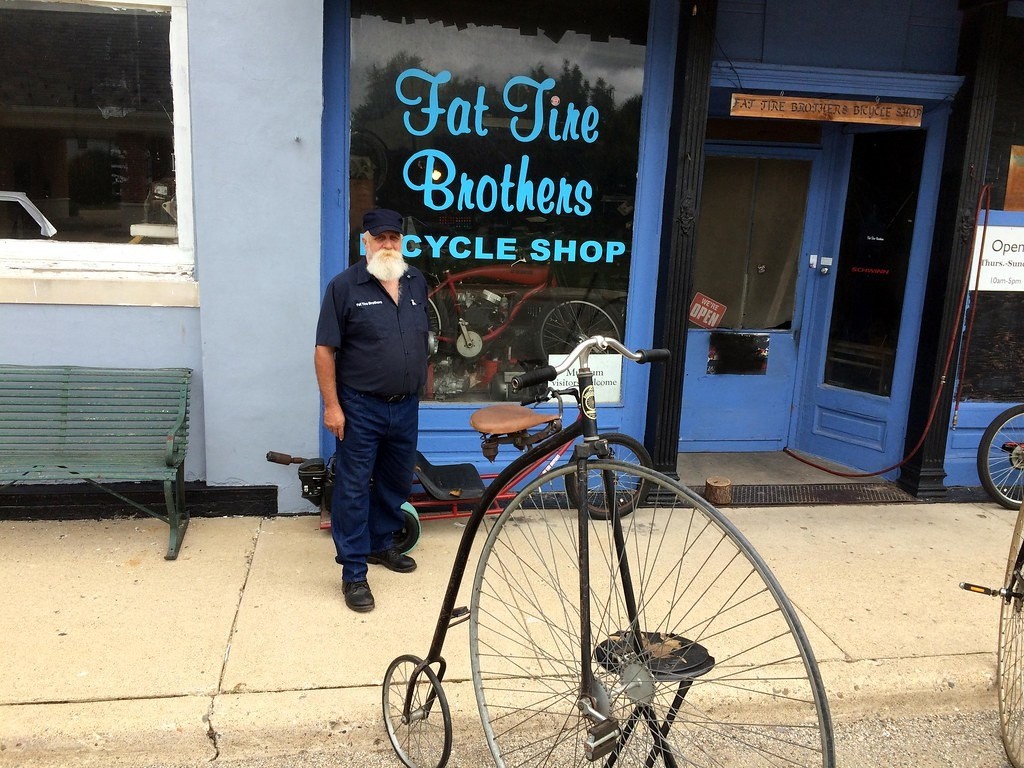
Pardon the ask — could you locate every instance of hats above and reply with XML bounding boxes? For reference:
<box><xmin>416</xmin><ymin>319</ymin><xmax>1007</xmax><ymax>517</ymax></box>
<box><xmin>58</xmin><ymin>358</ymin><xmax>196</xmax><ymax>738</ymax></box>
<box><xmin>362</xmin><ymin>209</ymin><xmax>404</xmax><ymax>236</ymax></box>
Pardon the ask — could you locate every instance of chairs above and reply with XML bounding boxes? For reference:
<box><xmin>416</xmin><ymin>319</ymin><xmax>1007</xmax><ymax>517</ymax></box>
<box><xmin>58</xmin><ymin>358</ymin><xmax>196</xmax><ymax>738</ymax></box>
<box><xmin>415</xmin><ymin>452</ymin><xmax>486</xmax><ymax>500</ymax></box>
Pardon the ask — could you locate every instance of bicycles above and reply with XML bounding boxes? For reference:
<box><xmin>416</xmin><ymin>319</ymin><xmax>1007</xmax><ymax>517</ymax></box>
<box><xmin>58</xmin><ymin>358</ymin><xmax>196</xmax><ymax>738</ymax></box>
<box><xmin>262</xmin><ymin>387</ymin><xmax>652</xmax><ymax>555</ymax></box>
<box><xmin>960</xmin><ymin>503</ymin><xmax>1024</xmax><ymax>768</ymax></box>
<box><xmin>382</xmin><ymin>334</ymin><xmax>834</xmax><ymax>768</ymax></box>
<box><xmin>976</xmin><ymin>406</ymin><xmax>1024</xmax><ymax>507</ymax></box>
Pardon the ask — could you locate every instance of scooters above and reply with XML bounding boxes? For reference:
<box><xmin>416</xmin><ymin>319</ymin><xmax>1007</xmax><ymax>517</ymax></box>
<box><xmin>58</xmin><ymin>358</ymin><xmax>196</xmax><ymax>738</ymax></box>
<box><xmin>418</xmin><ymin>224</ymin><xmax>624</xmax><ymax>373</ymax></box>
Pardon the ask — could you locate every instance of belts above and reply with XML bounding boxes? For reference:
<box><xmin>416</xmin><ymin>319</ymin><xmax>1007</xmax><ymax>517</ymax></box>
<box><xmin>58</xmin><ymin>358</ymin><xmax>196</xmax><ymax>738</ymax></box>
<box><xmin>346</xmin><ymin>384</ymin><xmax>416</xmax><ymax>403</ymax></box>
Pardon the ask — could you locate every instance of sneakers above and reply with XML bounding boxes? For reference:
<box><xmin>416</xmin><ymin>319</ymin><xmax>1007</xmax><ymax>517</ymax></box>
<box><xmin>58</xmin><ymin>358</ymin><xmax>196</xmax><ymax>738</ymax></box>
<box><xmin>341</xmin><ymin>579</ymin><xmax>375</xmax><ymax>611</ymax></box>
<box><xmin>367</xmin><ymin>548</ymin><xmax>417</xmax><ymax>573</ymax></box>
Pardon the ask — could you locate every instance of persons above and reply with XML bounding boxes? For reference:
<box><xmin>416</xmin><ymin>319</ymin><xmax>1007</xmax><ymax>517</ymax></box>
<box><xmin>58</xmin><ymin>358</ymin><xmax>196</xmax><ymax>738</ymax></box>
<box><xmin>314</xmin><ymin>209</ymin><xmax>430</xmax><ymax>613</ymax></box>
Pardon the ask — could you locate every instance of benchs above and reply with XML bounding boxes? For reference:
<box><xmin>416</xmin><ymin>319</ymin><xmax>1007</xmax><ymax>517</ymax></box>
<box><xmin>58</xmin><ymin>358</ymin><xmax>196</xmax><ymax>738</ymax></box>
<box><xmin>0</xmin><ymin>363</ymin><xmax>194</xmax><ymax>559</ymax></box>
